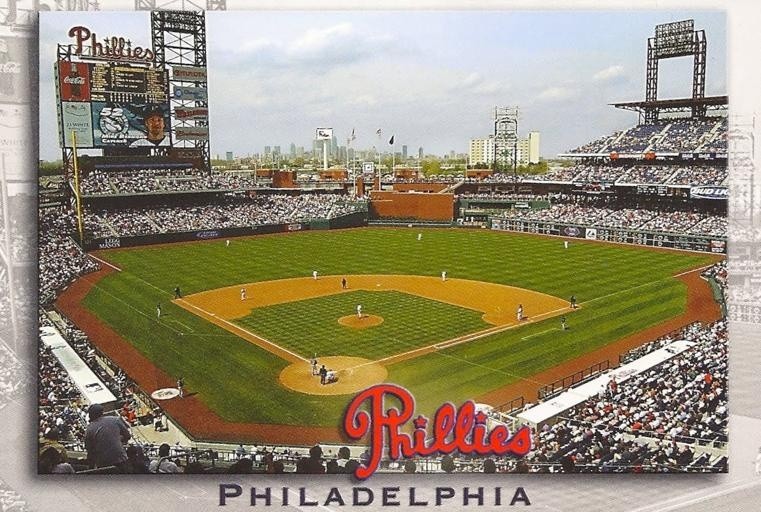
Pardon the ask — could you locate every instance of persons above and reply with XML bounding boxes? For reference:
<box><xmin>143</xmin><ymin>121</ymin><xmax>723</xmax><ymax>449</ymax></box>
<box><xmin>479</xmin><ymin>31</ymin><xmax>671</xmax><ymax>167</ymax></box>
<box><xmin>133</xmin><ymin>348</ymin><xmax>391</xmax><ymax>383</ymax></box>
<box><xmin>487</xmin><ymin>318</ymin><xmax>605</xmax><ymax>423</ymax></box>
<box><xmin>129</xmin><ymin>105</ymin><xmax>171</xmax><ymax>150</ymax></box>
<box><xmin>38</xmin><ymin>116</ymin><xmax>728</xmax><ymax>476</ymax></box>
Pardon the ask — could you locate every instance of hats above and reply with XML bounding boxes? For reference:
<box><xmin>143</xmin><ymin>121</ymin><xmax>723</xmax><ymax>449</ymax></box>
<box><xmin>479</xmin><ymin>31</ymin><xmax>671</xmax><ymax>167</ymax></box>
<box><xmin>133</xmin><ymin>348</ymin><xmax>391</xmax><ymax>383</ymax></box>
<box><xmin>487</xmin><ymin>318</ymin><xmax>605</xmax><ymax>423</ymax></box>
<box><xmin>142</xmin><ymin>103</ymin><xmax>164</xmax><ymax>119</ymax></box>
